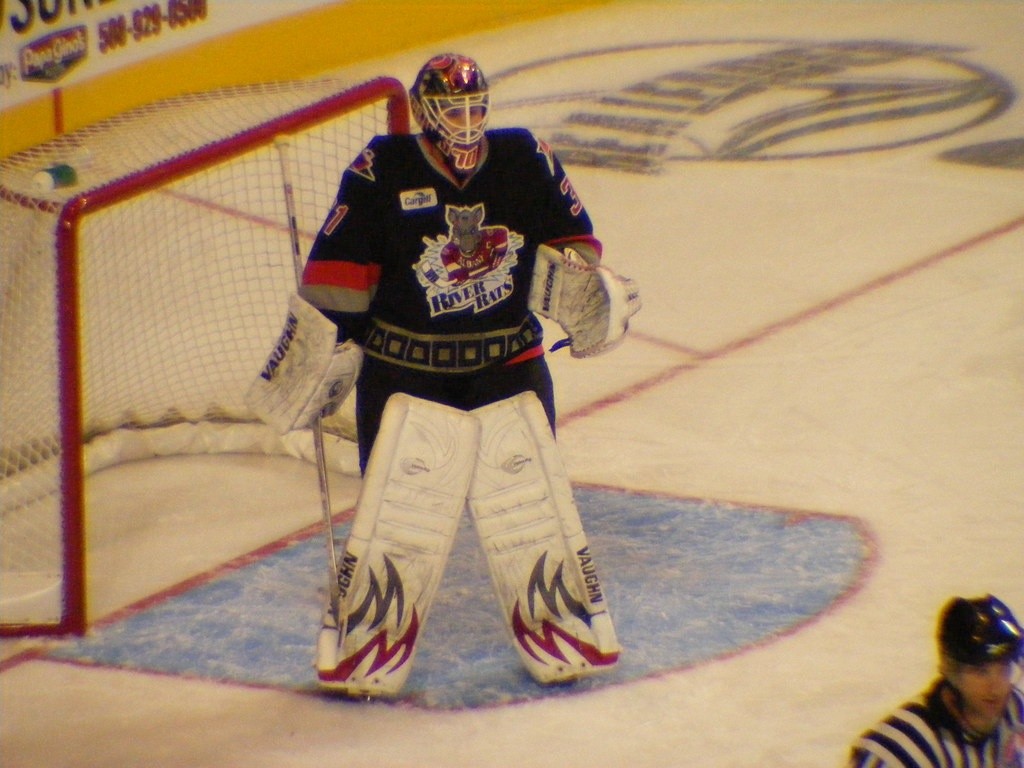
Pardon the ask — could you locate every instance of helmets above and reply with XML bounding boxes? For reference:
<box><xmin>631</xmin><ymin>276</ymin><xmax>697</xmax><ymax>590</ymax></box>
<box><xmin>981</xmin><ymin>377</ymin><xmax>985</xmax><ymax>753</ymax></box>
<box><xmin>937</xmin><ymin>591</ymin><xmax>1024</xmax><ymax>666</ymax></box>
<box><xmin>409</xmin><ymin>53</ymin><xmax>490</xmax><ymax>174</ymax></box>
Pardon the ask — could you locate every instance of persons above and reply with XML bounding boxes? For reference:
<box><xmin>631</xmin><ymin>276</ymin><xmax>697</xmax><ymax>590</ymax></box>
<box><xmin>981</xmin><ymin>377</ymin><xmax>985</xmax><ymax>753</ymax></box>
<box><xmin>847</xmin><ymin>593</ymin><xmax>1023</xmax><ymax>768</ymax></box>
<box><xmin>245</xmin><ymin>55</ymin><xmax>642</xmax><ymax>696</ymax></box>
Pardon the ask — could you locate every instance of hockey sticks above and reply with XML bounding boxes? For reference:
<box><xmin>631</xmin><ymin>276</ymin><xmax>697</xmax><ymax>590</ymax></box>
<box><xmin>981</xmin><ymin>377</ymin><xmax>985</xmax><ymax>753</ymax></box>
<box><xmin>274</xmin><ymin>134</ymin><xmax>344</xmax><ymax>641</ymax></box>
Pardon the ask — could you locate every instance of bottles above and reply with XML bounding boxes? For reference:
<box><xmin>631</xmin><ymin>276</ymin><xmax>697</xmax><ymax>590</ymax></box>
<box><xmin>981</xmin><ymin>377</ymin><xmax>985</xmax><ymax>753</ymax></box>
<box><xmin>35</xmin><ymin>164</ymin><xmax>78</xmax><ymax>191</ymax></box>
